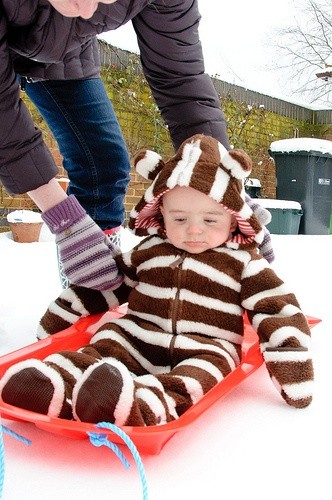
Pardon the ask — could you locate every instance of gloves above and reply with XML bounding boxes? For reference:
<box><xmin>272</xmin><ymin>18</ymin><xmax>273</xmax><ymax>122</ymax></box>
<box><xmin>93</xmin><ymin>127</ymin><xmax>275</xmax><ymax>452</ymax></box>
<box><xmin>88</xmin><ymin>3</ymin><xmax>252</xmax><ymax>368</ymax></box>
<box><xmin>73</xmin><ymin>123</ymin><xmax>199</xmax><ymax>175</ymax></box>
<box><xmin>243</xmin><ymin>193</ymin><xmax>275</xmax><ymax>263</ymax></box>
<box><xmin>43</xmin><ymin>194</ymin><xmax>124</xmax><ymax>292</ymax></box>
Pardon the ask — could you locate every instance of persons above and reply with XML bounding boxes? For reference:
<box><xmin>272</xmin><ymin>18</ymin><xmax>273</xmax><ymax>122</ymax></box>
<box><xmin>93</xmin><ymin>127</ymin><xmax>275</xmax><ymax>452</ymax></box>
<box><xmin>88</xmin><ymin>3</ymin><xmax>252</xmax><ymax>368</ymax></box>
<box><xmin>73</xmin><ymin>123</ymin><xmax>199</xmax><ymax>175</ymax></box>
<box><xmin>0</xmin><ymin>134</ymin><xmax>314</xmax><ymax>426</ymax></box>
<box><xmin>0</xmin><ymin>0</ymin><xmax>275</xmax><ymax>293</ymax></box>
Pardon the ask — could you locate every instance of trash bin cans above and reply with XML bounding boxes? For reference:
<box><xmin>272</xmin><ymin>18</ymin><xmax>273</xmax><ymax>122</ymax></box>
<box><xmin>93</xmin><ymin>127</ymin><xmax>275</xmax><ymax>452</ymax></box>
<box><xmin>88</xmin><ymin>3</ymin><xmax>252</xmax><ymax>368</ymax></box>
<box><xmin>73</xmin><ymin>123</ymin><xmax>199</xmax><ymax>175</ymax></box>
<box><xmin>250</xmin><ymin>199</ymin><xmax>303</xmax><ymax>236</ymax></box>
<box><xmin>268</xmin><ymin>138</ymin><xmax>332</xmax><ymax>235</ymax></box>
